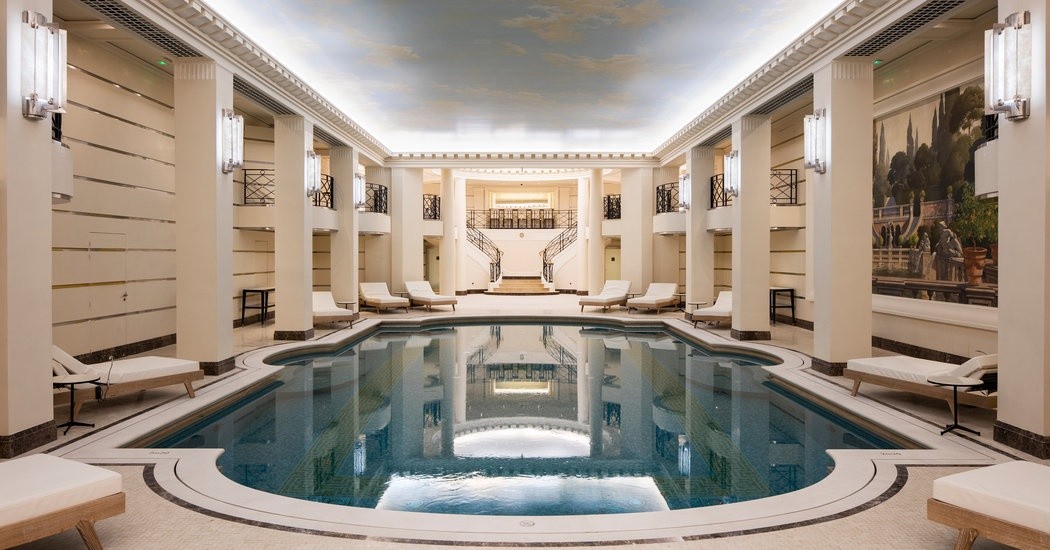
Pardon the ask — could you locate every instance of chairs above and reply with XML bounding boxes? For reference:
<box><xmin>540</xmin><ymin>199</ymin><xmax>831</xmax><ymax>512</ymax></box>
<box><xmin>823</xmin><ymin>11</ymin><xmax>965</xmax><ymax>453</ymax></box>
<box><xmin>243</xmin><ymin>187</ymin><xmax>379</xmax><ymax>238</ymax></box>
<box><xmin>0</xmin><ymin>452</ymin><xmax>127</xmax><ymax>550</ymax></box>
<box><xmin>313</xmin><ymin>348</ymin><xmax>358</xmax><ymax>368</ymax></box>
<box><xmin>404</xmin><ymin>280</ymin><xmax>458</xmax><ymax>312</ymax></box>
<box><xmin>50</xmin><ymin>343</ymin><xmax>205</xmax><ymax>423</ymax></box>
<box><xmin>928</xmin><ymin>460</ymin><xmax>1050</xmax><ymax>550</ymax></box>
<box><xmin>578</xmin><ymin>326</ymin><xmax>633</xmax><ymax>350</ymax></box>
<box><xmin>625</xmin><ymin>332</ymin><xmax>679</xmax><ymax>351</ymax></box>
<box><xmin>843</xmin><ymin>352</ymin><xmax>997</xmax><ymax>424</ymax></box>
<box><xmin>579</xmin><ymin>280</ymin><xmax>632</xmax><ymax>313</ymax></box>
<box><xmin>403</xmin><ymin>327</ymin><xmax>457</xmax><ymax>348</ymax></box>
<box><xmin>692</xmin><ymin>290</ymin><xmax>732</xmax><ymax>329</ymax></box>
<box><xmin>312</xmin><ymin>291</ymin><xmax>355</xmax><ymax>329</ymax></box>
<box><xmin>627</xmin><ymin>283</ymin><xmax>679</xmax><ymax>315</ymax></box>
<box><xmin>358</xmin><ymin>282</ymin><xmax>410</xmax><ymax>314</ymax></box>
<box><xmin>688</xmin><ymin>349</ymin><xmax>734</xmax><ymax>369</ymax></box>
<box><xmin>358</xmin><ymin>332</ymin><xmax>418</xmax><ymax>352</ymax></box>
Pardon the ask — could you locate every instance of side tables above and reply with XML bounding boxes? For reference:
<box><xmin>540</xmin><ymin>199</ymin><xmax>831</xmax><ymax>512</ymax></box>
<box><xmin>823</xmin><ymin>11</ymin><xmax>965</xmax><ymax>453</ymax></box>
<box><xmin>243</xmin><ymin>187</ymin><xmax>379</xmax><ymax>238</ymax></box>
<box><xmin>687</xmin><ymin>301</ymin><xmax>707</xmax><ymax>328</ymax></box>
<box><xmin>672</xmin><ymin>293</ymin><xmax>686</xmax><ymax>313</ymax></box>
<box><xmin>336</xmin><ymin>301</ymin><xmax>357</xmax><ymax>323</ymax></box>
<box><xmin>53</xmin><ymin>373</ymin><xmax>102</xmax><ymax>436</ymax></box>
<box><xmin>928</xmin><ymin>374</ymin><xmax>984</xmax><ymax>437</ymax></box>
<box><xmin>626</xmin><ymin>292</ymin><xmax>642</xmax><ymax>311</ymax></box>
<box><xmin>392</xmin><ymin>291</ymin><xmax>411</xmax><ymax>311</ymax></box>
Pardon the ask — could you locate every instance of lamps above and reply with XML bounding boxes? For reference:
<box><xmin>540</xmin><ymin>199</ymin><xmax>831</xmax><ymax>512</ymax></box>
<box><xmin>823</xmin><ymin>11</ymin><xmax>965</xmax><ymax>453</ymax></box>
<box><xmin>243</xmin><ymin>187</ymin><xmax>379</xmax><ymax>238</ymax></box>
<box><xmin>676</xmin><ymin>435</ymin><xmax>689</xmax><ymax>477</ymax></box>
<box><xmin>355</xmin><ymin>172</ymin><xmax>365</xmax><ymax>209</ymax></box>
<box><xmin>21</xmin><ymin>8</ymin><xmax>67</xmax><ymax>122</ymax></box>
<box><xmin>983</xmin><ymin>9</ymin><xmax>1030</xmax><ymax>123</ymax></box>
<box><xmin>723</xmin><ymin>150</ymin><xmax>739</xmax><ymax>199</ymax></box>
<box><xmin>221</xmin><ymin>107</ymin><xmax>245</xmax><ymax>174</ymax></box>
<box><xmin>802</xmin><ymin>107</ymin><xmax>827</xmax><ymax>175</ymax></box>
<box><xmin>353</xmin><ymin>440</ymin><xmax>364</xmax><ymax>478</ymax></box>
<box><xmin>678</xmin><ymin>172</ymin><xmax>691</xmax><ymax>210</ymax></box>
<box><xmin>307</xmin><ymin>150</ymin><xmax>321</xmax><ymax>197</ymax></box>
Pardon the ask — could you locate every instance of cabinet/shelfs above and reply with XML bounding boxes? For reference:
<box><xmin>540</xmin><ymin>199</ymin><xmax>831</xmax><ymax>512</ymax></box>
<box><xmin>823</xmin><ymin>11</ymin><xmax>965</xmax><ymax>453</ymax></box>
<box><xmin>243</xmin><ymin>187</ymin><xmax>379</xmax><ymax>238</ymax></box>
<box><xmin>769</xmin><ymin>286</ymin><xmax>795</xmax><ymax>325</ymax></box>
<box><xmin>240</xmin><ymin>287</ymin><xmax>276</xmax><ymax>325</ymax></box>
